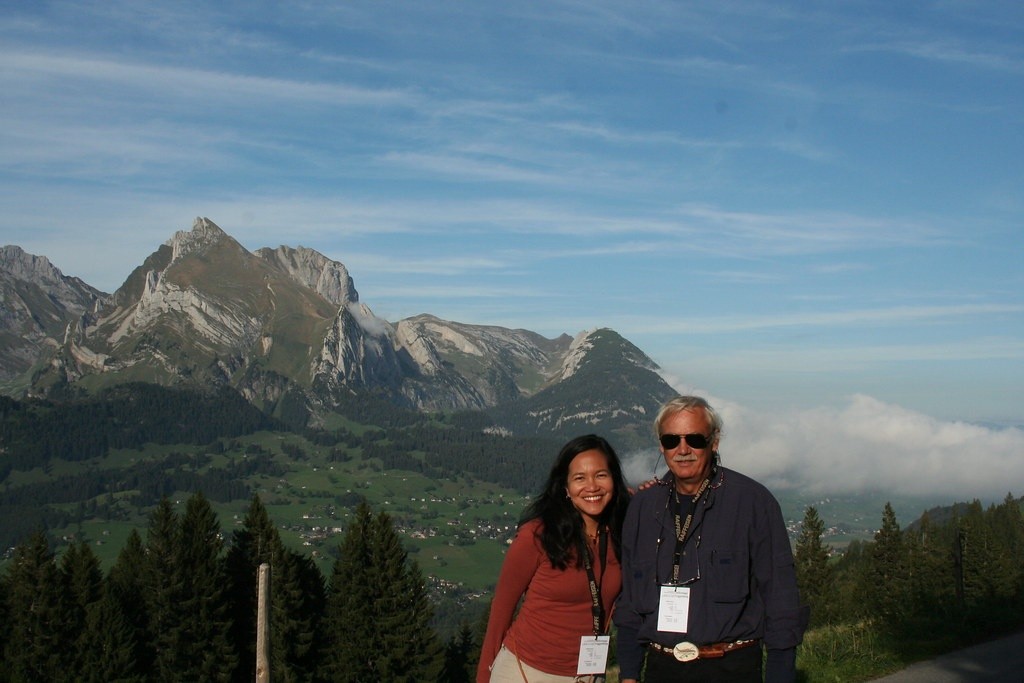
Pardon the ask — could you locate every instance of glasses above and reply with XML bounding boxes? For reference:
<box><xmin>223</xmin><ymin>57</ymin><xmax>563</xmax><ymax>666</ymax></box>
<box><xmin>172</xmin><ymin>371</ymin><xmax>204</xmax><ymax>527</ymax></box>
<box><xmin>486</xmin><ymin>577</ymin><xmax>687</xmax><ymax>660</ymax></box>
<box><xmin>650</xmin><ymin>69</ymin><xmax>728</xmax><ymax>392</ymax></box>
<box><xmin>658</xmin><ymin>430</ymin><xmax>715</xmax><ymax>450</ymax></box>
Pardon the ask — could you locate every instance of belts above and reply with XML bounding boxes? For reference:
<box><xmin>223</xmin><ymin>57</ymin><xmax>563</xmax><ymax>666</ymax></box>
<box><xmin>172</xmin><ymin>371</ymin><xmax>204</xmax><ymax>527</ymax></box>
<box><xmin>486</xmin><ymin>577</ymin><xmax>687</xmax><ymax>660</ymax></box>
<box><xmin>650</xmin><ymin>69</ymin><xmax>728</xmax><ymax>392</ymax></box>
<box><xmin>647</xmin><ymin>639</ymin><xmax>760</xmax><ymax>662</ymax></box>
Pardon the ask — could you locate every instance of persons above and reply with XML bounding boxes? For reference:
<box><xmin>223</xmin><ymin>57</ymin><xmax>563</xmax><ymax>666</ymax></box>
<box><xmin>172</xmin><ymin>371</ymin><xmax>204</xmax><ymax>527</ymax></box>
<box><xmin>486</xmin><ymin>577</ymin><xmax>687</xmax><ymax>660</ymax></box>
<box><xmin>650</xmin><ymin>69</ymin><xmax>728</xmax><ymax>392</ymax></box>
<box><xmin>475</xmin><ymin>435</ymin><xmax>655</xmax><ymax>683</ymax></box>
<box><xmin>612</xmin><ymin>394</ymin><xmax>811</xmax><ymax>683</ymax></box>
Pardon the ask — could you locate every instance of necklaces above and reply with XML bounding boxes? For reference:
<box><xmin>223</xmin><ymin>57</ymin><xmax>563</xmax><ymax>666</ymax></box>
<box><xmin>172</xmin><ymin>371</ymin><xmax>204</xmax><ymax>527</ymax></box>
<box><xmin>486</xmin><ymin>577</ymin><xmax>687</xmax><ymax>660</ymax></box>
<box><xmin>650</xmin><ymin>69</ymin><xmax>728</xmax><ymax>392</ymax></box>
<box><xmin>588</xmin><ymin>531</ymin><xmax>599</xmax><ymax>538</ymax></box>
